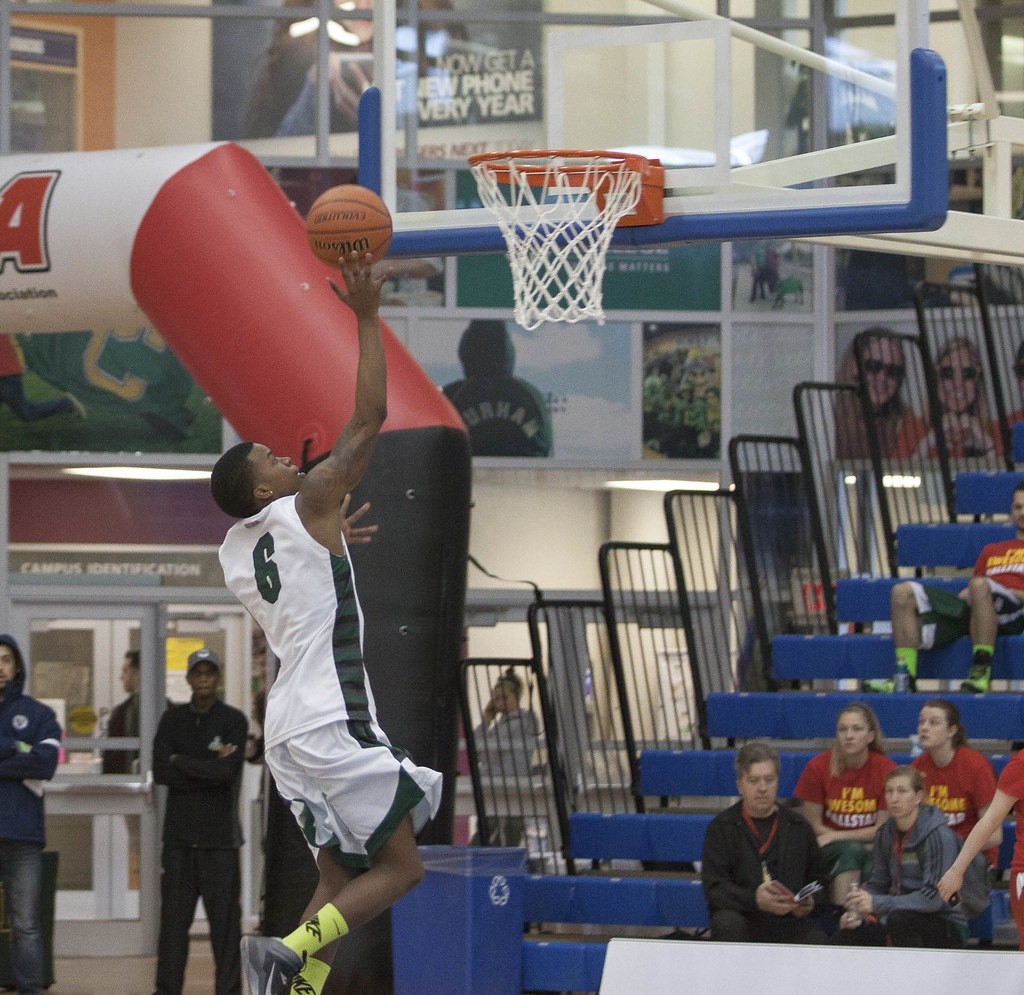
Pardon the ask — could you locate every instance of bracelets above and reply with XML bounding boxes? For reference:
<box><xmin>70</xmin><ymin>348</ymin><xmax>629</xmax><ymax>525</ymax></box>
<box><xmin>983</xmin><ymin>446</ymin><xmax>996</xmax><ymax>454</ymax></box>
<box><xmin>387</xmin><ymin>261</ymin><xmax>394</xmax><ymax>280</ymax></box>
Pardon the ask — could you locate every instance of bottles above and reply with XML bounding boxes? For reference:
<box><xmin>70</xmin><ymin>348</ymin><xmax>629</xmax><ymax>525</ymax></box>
<box><xmin>894</xmin><ymin>656</ymin><xmax>909</xmax><ymax>695</ymax></box>
<box><xmin>846</xmin><ymin>883</ymin><xmax>863</xmax><ymax>928</ymax></box>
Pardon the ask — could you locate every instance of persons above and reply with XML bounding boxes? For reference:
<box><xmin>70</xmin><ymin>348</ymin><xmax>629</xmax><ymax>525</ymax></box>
<box><xmin>703</xmin><ymin>484</ymin><xmax>1024</xmax><ymax>949</ymax></box>
<box><xmin>0</xmin><ymin>333</ymin><xmax>86</xmax><ymax>422</ymax></box>
<box><xmin>210</xmin><ymin>251</ymin><xmax>445</xmax><ymax>995</ymax></box>
<box><xmin>248</xmin><ymin>0</ymin><xmax>479</xmax><ymax>139</ymax></box>
<box><xmin>348</xmin><ymin>170</ymin><xmax>442</xmax><ymax>305</ymax></box>
<box><xmin>1</xmin><ymin>632</ymin><xmax>536</xmax><ymax>995</ymax></box>
<box><xmin>644</xmin><ymin>239</ymin><xmax>1024</xmax><ymax>461</ymax></box>
<box><xmin>440</xmin><ymin>319</ymin><xmax>556</xmax><ymax>459</ymax></box>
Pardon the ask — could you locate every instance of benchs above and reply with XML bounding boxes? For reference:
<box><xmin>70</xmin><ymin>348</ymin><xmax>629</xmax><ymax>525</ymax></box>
<box><xmin>520</xmin><ymin>419</ymin><xmax>1024</xmax><ymax>995</ymax></box>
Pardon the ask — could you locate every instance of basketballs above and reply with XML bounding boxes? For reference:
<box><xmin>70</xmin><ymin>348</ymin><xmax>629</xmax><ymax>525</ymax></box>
<box><xmin>306</xmin><ymin>184</ymin><xmax>392</xmax><ymax>270</ymax></box>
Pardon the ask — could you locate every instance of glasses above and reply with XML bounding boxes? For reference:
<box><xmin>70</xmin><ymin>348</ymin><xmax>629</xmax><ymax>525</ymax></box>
<box><xmin>937</xmin><ymin>364</ymin><xmax>982</xmax><ymax>379</ymax></box>
<box><xmin>863</xmin><ymin>357</ymin><xmax>905</xmax><ymax>379</ymax></box>
<box><xmin>193</xmin><ymin>669</ymin><xmax>214</xmax><ymax>677</ymax></box>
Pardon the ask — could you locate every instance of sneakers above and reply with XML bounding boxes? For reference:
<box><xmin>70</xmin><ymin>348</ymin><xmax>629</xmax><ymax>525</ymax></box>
<box><xmin>961</xmin><ymin>649</ymin><xmax>994</xmax><ymax>693</ymax></box>
<box><xmin>239</xmin><ymin>932</ymin><xmax>301</xmax><ymax>995</ymax></box>
<box><xmin>861</xmin><ymin>663</ymin><xmax>916</xmax><ymax>693</ymax></box>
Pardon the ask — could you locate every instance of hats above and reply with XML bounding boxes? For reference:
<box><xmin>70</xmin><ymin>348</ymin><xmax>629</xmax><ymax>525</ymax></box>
<box><xmin>188</xmin><ymin>649</ymin><xmax>221</xmax><ymax>677</ymax></box>
